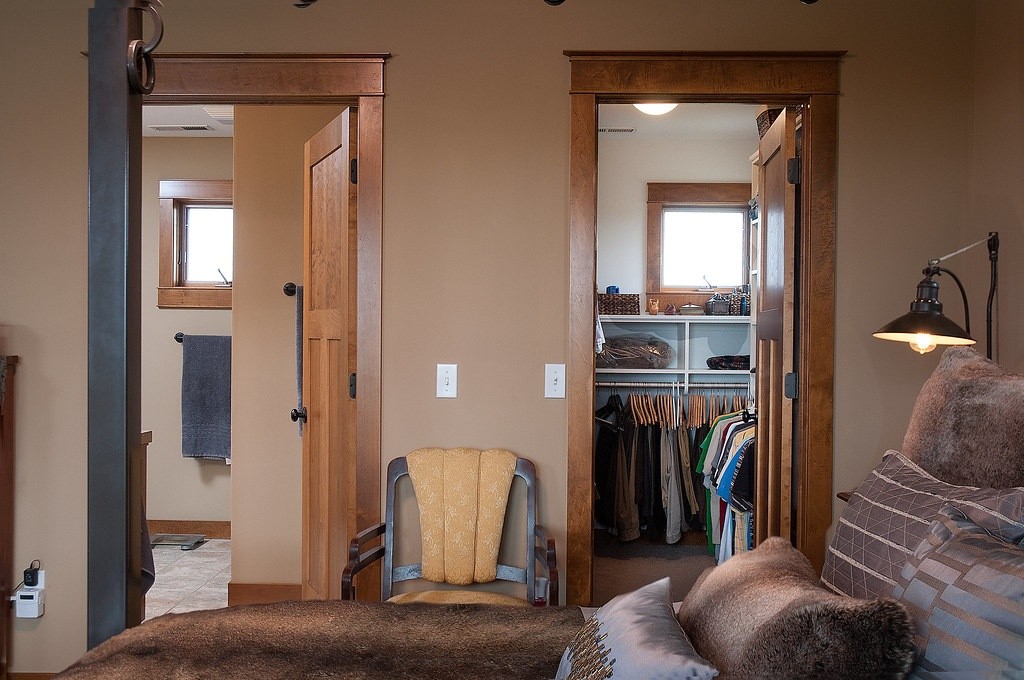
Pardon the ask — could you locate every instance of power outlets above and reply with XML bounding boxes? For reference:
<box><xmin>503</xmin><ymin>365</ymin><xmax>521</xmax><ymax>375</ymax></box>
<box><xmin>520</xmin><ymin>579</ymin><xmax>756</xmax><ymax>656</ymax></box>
<box><xmin>24</xmin><ymin>570</ymin><xmax>45</xmax><ymax>590</ymax></box>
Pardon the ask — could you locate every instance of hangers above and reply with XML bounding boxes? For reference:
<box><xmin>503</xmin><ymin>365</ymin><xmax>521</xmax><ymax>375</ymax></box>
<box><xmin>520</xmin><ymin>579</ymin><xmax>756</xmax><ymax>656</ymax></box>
<box><xmin>623</xmin><ymin>380</ymin><xmax>750</xmax><ymax>431</ymax></box>
<box><xmin>595</xmin><ymin>381</ymin><xmax>625</xmax><ymax>433</ymax></box>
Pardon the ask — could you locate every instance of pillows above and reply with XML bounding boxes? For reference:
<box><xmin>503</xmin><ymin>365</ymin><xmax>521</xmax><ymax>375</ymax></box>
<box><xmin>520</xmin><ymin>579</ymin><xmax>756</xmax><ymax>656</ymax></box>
<box><xmin>901</xmin><ymin>346</ymin><xmax>1023</xmax><ymax>490</ymax></box>
<box><xmin>678</xmin><ymin>537</ymin><xmax>920</xmax><ymax>679</ymax></box>
<box><xmin>556</xmin><ymin>576</ymin><xmax>720</xmax><ymax>680</ymax></box>
<box><xmin>818</xmin><ymin>449</ymin><xmax>1023</xmax><ymax>680</ymax></box>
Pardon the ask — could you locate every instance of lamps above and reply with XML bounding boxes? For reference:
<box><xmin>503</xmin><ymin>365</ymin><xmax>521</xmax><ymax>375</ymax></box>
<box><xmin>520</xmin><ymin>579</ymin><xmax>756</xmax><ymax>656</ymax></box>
<box><xmin>870</xmin><ymin>232</ymin><xmax>1000</xmax><ymax>360</ymax></box>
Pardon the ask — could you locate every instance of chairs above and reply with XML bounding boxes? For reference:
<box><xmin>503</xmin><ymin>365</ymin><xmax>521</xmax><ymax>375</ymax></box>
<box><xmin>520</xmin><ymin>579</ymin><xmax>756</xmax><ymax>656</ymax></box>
<box><xmin>341</xmin><ymin>447</ymin><xmax>560</xmax><ymax>607</ymax></box>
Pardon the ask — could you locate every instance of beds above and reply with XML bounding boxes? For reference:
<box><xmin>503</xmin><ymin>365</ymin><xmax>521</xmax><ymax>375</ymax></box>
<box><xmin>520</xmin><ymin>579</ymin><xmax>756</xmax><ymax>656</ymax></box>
<box><xmin>51</xmin><ymin>604</ymin><xmax>685</xmax><ymax>680</ymax></box>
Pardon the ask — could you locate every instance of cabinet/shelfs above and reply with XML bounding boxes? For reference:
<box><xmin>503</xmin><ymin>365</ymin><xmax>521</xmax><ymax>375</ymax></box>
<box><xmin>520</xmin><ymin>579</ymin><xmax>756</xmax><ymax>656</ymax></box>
<box><xmin>596</xmin><ymin>315</ymin><xmax>751</xmax><ymax>395</ymax></box>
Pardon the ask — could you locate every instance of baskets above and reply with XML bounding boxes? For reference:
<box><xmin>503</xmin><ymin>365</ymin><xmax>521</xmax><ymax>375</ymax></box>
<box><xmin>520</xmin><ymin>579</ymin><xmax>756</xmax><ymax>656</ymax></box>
<box><xmin>597</xmin><ymin>293</ymin><xmax>640</xmax><ymax>315</ymax></box>
